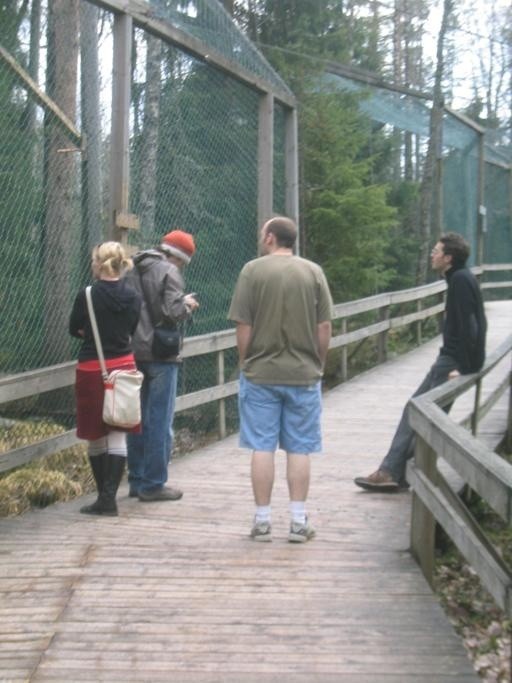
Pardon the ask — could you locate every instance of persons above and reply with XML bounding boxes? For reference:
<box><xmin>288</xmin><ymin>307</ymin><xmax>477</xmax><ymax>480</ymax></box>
<box><xmin>130</xmin><ymin>229</ymin><xmax>201</xmax><ymax>502</ymax></box>
<box><xmin>352</xmin><ymin>231</ymin><xmax>489</xmax><ymax>494</ymax></box>
<box><xmin>67</xmin><ymin>238</ymin><xmax>143</xmax><ymax>518</ymax></box>
<box><xmin>225</xmin><ymin>215</ymin><xmax>336</xmax><ymax>543</ymax></box>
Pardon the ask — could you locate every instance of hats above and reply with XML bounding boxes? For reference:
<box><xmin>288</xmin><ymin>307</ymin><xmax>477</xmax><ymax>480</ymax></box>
<box><xmin>159</xmin><ymin>230</ymin><xmax>195</xmax><ymax>265</ymax></box>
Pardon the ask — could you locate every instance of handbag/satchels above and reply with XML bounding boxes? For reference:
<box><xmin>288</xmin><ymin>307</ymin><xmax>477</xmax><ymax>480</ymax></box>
<box><xmin>102</xmin><ymin>369</ymin><xmax>144</xmax><ymax>428</ymax></box>
<box><xmin>152</xmin><ymin>329</ymin><xmax>179</xmax><ymax>359</ymax></box>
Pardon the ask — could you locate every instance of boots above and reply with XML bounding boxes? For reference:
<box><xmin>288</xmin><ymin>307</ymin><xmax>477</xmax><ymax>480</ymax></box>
<box><xmin>79</xmin><ymin>452</ymin><xmax>127</xmax><ymax>517</ymax></box>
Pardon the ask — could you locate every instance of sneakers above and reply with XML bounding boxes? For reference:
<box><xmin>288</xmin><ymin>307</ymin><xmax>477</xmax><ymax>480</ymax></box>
<box><xmin>250</xmin><ymin>522</ymin><xmax>273</xmax><ymax>542</ymax></box>
<box><xmin>287</xmin><ymin>514</ymin><xmax>317</xmax><ymax>543</ymax></box>
<box><xmin>353</xmin><ymin>470</ymin><xmax>408</xmax><ymax>492</ymax></box>
<box><xmin>140</xmin><ymin>485</ymin><xmax>184</xmax><ymax>501</ymax></box>
